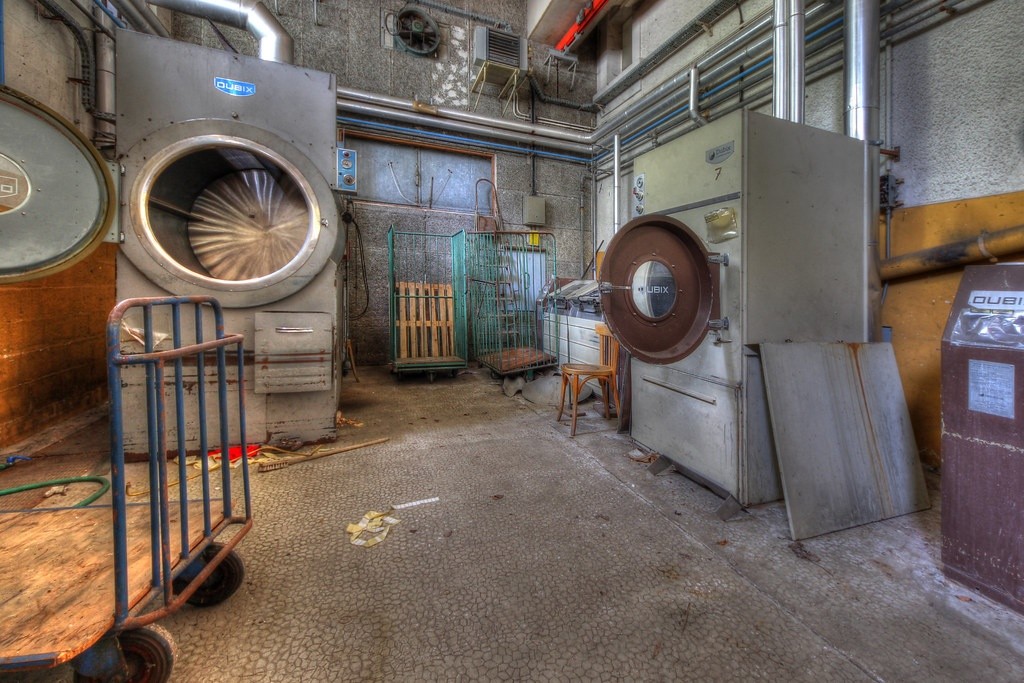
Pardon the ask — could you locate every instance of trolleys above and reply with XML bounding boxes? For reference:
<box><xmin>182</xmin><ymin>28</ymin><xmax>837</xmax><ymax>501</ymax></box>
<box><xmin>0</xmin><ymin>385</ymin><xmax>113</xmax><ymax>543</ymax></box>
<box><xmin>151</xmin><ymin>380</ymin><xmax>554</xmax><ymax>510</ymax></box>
<box><xmin>0</xmin><ymin>294</ymin><xmax>254</xmax><ymax>683</ymax></box>
<box><xmin>388</xmin><ymin>279</ymin><xmax>466</xmax><ymax>384</ymax></box>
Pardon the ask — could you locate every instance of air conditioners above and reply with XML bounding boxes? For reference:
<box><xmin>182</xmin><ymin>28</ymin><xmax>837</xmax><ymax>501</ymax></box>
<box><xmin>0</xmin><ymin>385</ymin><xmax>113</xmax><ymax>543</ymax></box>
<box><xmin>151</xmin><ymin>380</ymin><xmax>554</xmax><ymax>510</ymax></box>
<box><xmin>472</xmin><ymin>26</ymin><xmax>529</xmax><ymax>79</ymax></box>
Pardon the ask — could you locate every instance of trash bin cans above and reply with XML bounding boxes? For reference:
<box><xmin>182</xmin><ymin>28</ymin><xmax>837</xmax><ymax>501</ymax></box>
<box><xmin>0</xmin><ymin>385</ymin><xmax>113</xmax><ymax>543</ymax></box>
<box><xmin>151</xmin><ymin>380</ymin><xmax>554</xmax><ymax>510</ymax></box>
<box><xmin>941</xmin><ymin>265</ymin><xmax>1024</xmax><ymax>615</ymax></box>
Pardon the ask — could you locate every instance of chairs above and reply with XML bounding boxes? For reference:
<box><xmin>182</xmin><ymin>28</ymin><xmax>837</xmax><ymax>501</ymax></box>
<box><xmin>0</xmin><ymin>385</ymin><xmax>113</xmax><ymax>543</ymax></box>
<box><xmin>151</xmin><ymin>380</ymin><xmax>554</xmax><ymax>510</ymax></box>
<box><xmin>556</xmin><ymin>322</ymin><xmax>621</xmax><ymax>437</ymax></box>
